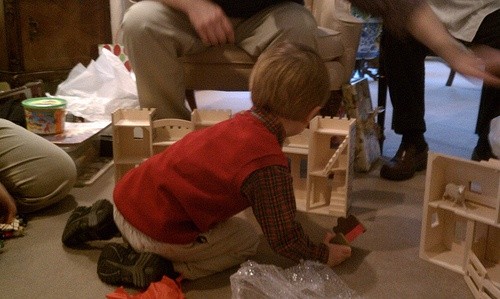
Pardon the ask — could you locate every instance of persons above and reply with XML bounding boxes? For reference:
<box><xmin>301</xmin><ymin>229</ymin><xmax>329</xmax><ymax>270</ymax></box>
<box><xmin>0</xmin><ymin>118</ymin><xmax>77</xmax><ymax>224</ymax></box>
<box><xmin>62</xmin><ymin>44</ymin><xmax>351</xmax><ymax>290</ymax></box>
<box><xmin>380</xmin><ymin>0</ymin><xmax>500</xmax><ymax>181</ymax></box>
<box><xmin>121</xmin><ymin>0</ymin><xmax>500</xmax><ymax>122</ymax></box>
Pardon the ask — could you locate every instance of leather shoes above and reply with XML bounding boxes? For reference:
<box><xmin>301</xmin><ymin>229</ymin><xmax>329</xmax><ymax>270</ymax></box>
<box><xmin>380</xmin><ymin>145</ymin><xmax>429</xmax><ymax>180</ymax></box>
<box><xmin>472</xmin><ymin>147</ymin><xmax>480</xmax><ymax>162</ymax></box>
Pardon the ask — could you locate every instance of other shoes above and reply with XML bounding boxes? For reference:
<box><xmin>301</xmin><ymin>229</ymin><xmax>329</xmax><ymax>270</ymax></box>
<box><xmin>96</xmin><ymin>242</ymin><xmax>175</xmax><ymax>288</ymax></box>
<box><xmin>61</xmin><ymin>199</ymin><xmax>121</xmax><ymax>245</ymax></box>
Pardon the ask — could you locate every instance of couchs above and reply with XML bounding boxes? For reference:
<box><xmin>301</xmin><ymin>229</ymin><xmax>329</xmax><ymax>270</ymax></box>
<box><xmin>110</xmin><ymin>0</ymin><xmax>362</xmax><ymax>113</ymax></box>
